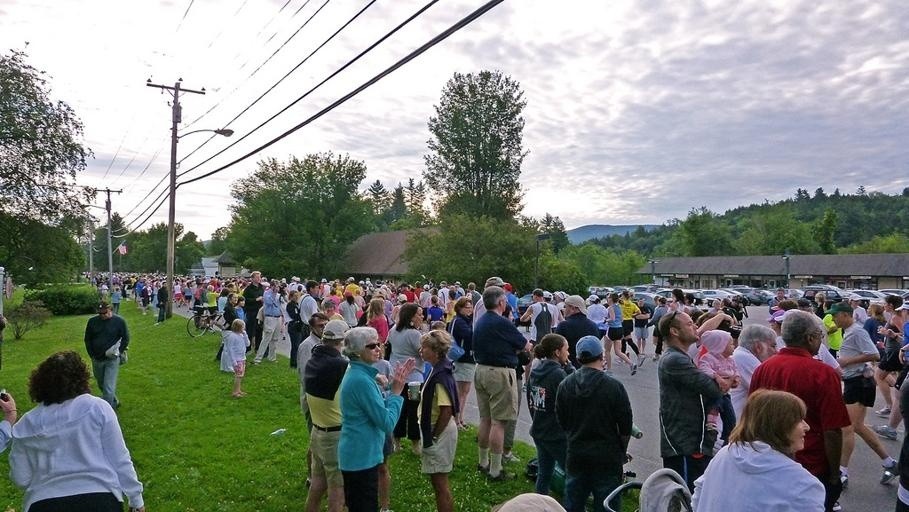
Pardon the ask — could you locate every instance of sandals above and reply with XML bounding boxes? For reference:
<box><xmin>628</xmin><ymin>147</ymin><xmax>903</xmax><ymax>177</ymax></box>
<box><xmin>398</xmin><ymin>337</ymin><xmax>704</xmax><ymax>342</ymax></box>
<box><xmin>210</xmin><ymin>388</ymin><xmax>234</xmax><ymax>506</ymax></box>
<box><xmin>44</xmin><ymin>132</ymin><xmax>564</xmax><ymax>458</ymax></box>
<box><xmin>231</xmin><ymin>392</ymin><xmax>243</xmax><ymax>397</ymax></box>
<box><xmin>238</xmin><ymin>391</ymin><xmax>248</xmax><ymax>395</ymax></box>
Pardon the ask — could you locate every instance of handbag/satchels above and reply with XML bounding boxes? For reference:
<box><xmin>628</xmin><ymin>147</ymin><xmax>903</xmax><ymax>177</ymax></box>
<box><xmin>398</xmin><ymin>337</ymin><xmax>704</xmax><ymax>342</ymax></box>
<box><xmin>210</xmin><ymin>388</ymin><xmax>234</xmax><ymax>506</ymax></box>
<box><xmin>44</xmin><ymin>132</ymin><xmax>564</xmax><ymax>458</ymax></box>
<box><xmin>439</xmin><ymin>307</ymin><xmax>445</xmax><ymax>314</ymax></box>
<box><xmin>292</xmin><ymin>319</ymin><xmax>303</xmax><ymax>332</ymax></box>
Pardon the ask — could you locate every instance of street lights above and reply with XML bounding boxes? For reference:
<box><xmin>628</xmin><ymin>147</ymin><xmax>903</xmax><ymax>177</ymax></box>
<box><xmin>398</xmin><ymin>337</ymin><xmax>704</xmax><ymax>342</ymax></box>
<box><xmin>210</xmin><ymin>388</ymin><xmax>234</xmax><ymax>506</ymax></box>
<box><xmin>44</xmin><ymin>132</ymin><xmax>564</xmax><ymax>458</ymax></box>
<box><xmin>164</xmin><ymin>128</ymin><xmax>234</xmax><ymax>319</ymax></box>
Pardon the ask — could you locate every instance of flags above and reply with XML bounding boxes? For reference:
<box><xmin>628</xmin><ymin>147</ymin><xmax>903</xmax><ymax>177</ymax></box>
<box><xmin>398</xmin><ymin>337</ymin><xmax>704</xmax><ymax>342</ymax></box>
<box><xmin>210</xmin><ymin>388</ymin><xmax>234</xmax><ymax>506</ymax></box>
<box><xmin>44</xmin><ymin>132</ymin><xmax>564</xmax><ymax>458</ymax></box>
<box><xmin>119</xmin><ymin>240</ymin><xmax>127</xmax><ymax>256</ymax></box>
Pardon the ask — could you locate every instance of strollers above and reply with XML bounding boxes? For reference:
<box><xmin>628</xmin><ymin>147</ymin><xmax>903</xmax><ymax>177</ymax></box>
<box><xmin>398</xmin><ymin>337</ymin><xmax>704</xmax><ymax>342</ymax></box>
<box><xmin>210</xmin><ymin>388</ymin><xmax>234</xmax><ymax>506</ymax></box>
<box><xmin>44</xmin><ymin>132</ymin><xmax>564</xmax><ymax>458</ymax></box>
<box><xmin>601</xmin><ymin>463</ymin><xmax>720</xmax><ymax>512</ymax></box>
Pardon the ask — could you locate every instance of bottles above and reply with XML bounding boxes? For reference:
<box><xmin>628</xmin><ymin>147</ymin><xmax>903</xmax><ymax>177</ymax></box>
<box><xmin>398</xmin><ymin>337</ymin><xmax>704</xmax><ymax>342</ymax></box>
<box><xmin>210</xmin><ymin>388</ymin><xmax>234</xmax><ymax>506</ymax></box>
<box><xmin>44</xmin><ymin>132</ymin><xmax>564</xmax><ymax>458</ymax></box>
<box><xmin>0</xmin><ymin>390</ymin><xmax>9</xmax><ymax>411</ymax></box>
<box><xmin>408</xmin><ymin>382</ymin><xmax>421</xmax><ymax>401</ymax></box>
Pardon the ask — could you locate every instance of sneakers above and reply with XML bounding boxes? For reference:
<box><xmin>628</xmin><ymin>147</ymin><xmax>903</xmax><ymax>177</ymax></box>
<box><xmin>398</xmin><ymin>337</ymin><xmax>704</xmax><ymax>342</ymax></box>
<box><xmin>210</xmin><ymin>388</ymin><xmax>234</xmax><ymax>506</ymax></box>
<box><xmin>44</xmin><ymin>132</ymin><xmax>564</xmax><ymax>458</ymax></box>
<box><xmin>282</xmin><ymin>334</ymin><xmax>286</xmax><ymax>340</ymax></box>
<box><xmin>246</xmin><ymin>350</ymin><xmax>252</xmax><ymax>356</ymax></box>
<box><xmin>253</xmin><ymin>361</ymin><xmax>260</xmax><ymax>366</ymax></box>
<box><xmin>653</xmin><ymin>355</ymin><xmax>659</xmax><ymax>362</ymax></box>
<box><xmin>502</xmin><ymin>453</ymin><xmax>521</xmax><ymax>462</ymax></box>
<box><xmin>637</xmin><ymin>355</ymin><xmax>645</xmax><ymax>368</ymax></box>
<box><xmin>603</xmin><ymin>370</ymin><xmax>614</xmax><ymax>378</ymax></box>
<box><xmin>267</xmin><ymin>359</ymin><xmax>279</xmax><ymax>363</ymax></box>
<box><xmin>411</xmin><ymin>446</ymin><xmax>422</xmax><ymax>456</ymax></box>
<box><xmin>881</xmin><ymin>461</ymin><xmax>899</xmax><ymax>485</ymax></box>
<box><xmin>872</xmin><ymin>424</ymin><xmax>897</xmax><ymax>441</ymax></box>
<box><xmin>877</xmin><ymin>409</ymin><xmax>892</xmax><ymax>416</ymax></box>
<box><xmin>630</xmin><ymin>362</ymin><xmax>638</xmax><ymax>376</ymax></box>
<box><xmin>839</xmin><ymin>470</ymin><xmax>849</xmax><ymax>490</ymax></box>
<box><xmin>706</xmin><ymin>422</ymin><xmax>718</xmax><ymax>435</ymax></box>
<box><xmin>394</xmin><ymin>445</ymin><xmax>403</xmax><ymax>452</ymax></box>
<box><xmin>614</xmin><ymin>356</ymin><xmax>624</xmax><ymax>363</ymax></box>
<box><xmin>642</xmin><ymin>350</ymin><xmax>645</xmax><ymax>355</ymax></box>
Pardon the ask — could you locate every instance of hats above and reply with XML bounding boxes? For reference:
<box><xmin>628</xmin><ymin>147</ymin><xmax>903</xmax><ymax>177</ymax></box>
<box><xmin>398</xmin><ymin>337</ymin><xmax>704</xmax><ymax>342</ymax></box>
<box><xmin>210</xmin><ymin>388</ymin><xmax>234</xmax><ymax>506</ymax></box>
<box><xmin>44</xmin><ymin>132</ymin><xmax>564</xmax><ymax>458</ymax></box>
<box><xmin>282</xmin><ymin>278</ymin><xmax>286</xmax><ymax>284</ymax></box>
<box><xmin>381</xmin><ymin>284</ymin><xmax>387</xmax><ymax>289</ymax></box>
<box><xmin>585</xmin><ymin>298</ymin><xmax>590</xmax><ymax>305</ymax></box>
<box><xmin>484</xmin><ymin>277</ymin><xmax>508</xmax><ymax>288</ymax></box>
<box><xmin>797</xmin><ymin>298</ymin><xmax>812</xmax><ymax>308</ymax></box>
<box><xmin>270</xmin><ymin>278</ymin><xmax>275</xmax><ymax>282</ymax></box>
<box><xmin>262</xmin><ymin>277</ymin><xmax>267</xmax><ymax>281</ymax></box>
<box><xmin>396</xmin><ymin>294</ymin><xmax>407</xmax><ymax>302</ymax></box>
<box><xmin>823</xmin><ymin>303</ymin><xmax>853</xmax><ymax>314</ymax></box>
<box><xmin>503</xmin><ymin>283</ymin><xmax>513</xmax><ymax>293</ymax></box>
<box><xmin>771</xmin><ymin>304</ymin><xmax>785</xmax><ymax>311</ymax></box>
<box><xmin>348</xmin><ymin>276</ymin><xmax>355</xmax><ymax>282</ymax></box>
<box><xmin>261</xmin><ymin>281</ymin><xmax>270</xmax><ymax>287</ymax></box>
<box><xmin>321</xmin><ymin>278</ymin><xmax>327</xmax><ymax>283</ymax></box>
<box><xmin>453</xmin><ymin>281</ymin><xmax>462</xmax><ymax>286</ymax></box>
<box><xmin>565</xmin><ymin>294</ymin><xmax>589</xmax><ymax>316</ymax></box>
<box><xmin>345</xmin><ymin>290</ymin><xmax>352</xmax><ymax>297</ymax></box>
<box><xmin>366</xmin><ymin>278</ymin><xmax>370</xmax><ymax>281</ymax></box>
<box><xmin>895</xmin><ymin>298</ymin><xmax>909</xmax><ymax>311</ymax></box>
<box><xmin>440</xmin><ymin>281</ymin><xmax>447</xmax><ymax>284</ymax></box>
<box><xmin>97</xmin><ymin>300</ymin><xmax>111</xmax><ymax>309</ymax></box>
<box><xmin>587</xmin><ymin>294</ymin><xmax>599</xmax><ymax>302</ymax></box>
<box><xmin>542</xmin><ymin>291</ymin><xmax>553</xmax><ymax>298</ymax></box>
<box><xmin>556</xmin><ymin>291</ymin><xmax>566</xmax><ymax>300</ymax></box>
<box><xmin>322</xmin><ymin>319</ymin><xmax>349</xmax><ymax>340</ymax></box>
<box><xmin>576</xmin><ymin>335</ymin><xmax>604</xmax><ymax>359</ymax></box>
<box><xmin>291</xmin><ymin>276</ymin><xmax>296</xmax><ymax>280</ymax></box>
<box><xmin>295</xmin><ymin>277</ymin><xmax>301</xmax><ymax>282</ymax></box>
<box><xmin>600</xmin><ymin>299</ymin><xmax>607</xmax><ymax>306</ymax></box>
<box><xmin>365</xmin><ymin>281</ymin><xmax>371</xmax><ymax>285</ymax></box>
<box><xmin>552</xmin><ymin>291</ymin><xmax>559</xmax><ymax>296</ymax></box>
<box><xmin>359</xmin><ymin>280</ymin><xmax>364</xmax><ymax>285</ymax></box>
<box><xmin>423</xmin><ymin>284</ymin><xmax>430</xmax><ymax>290</ymax></box>
<box><xmin>638</xmin><ymin>298</ymin><xmax>646</xmax><ymax>302</ymax></box>
<box><xmin>377</xmin><ymin>291</ymin><xmax>385</xmax><ymax>297</ymax></box>
<box><xmin>376</xmin><ymin>284</ymin><xmax>381</xmax><ymax>287</ymax></box>
<box><xmin>770</xmin><ymin>308</ymin><xmax>785</xmax><ymax>324</ymax></box>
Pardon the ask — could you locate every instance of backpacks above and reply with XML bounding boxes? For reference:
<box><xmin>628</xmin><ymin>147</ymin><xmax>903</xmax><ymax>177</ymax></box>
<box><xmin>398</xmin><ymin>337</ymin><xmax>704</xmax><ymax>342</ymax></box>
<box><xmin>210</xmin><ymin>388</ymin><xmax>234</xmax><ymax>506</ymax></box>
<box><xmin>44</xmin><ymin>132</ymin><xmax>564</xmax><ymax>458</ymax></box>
<box><xmin>472</xmin><ymin>291</ymin><xmax>481</xmax><ymax>308</ymax></box>
<box><xmin>447</xmin><ymin>319</ymin><xmax>465</xmax><ymax>362</ymax></box>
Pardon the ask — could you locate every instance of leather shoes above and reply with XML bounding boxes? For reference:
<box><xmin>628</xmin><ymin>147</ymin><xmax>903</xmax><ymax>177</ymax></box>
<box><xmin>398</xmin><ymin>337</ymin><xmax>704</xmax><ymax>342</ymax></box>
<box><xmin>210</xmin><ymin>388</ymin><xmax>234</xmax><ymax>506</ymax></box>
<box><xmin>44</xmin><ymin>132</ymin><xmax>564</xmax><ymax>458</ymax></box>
<box><xmin>477</xmin><ymin>460</ymin><xmax>490</xmax><ymax>473</ymax></box>
<box><xmin>487</xmin><ymin>469</ymin><xmax>517</xmax><ymax>482</ymax></box>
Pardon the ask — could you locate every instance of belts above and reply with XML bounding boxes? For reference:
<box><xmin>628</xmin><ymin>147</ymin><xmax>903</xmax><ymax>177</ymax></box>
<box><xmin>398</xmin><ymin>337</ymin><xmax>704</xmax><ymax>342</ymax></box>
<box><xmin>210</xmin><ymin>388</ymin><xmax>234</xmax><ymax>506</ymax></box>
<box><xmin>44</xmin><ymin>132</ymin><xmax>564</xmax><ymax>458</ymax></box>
<box><xmin>264</xmin><ymin>315</ymin><xmax>282</xmax><ymax>318</ymax></box>
<box><xmin>493</xmin><ymin>364</ymin><xmax>517</xmax><ymax>370</ymax></box>
<box><xmin>311</xmin><ymin>423</ymin><xmax>341</xmax><ymax>433</ymax></box>
<box><xmin>302</xmin><ymin>324</ymin><xmax>309</xmax><ymax>326</ymax></box>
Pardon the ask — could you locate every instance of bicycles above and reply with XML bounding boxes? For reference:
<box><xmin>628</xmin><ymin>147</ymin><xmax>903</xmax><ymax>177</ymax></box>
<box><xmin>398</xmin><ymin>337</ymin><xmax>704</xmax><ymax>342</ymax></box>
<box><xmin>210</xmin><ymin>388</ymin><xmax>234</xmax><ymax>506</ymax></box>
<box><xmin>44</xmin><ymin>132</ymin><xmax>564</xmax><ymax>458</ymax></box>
<box><xmin>185</xmin><ymin>296</ymin><xmax>235</xmax><ymax>338</ymax></box>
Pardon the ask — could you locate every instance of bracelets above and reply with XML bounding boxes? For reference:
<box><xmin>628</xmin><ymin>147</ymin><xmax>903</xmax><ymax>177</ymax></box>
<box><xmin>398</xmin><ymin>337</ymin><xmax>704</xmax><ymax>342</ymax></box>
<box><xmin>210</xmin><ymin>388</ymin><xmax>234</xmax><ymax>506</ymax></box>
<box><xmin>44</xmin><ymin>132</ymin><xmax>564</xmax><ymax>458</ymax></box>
<box><xmin>6</xmin><ymin>409</ymin><xmax>18</xmax><ymax>413</ymax></box>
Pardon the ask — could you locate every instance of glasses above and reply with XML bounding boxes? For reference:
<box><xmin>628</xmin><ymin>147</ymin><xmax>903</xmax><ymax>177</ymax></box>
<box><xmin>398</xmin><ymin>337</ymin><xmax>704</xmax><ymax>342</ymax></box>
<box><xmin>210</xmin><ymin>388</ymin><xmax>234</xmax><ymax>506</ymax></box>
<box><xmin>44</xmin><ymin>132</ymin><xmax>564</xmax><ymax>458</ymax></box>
<box><xmin>364</xmin><ymin>343</ymin><xmax>380</xmax><ymax>350</ymax></box>
<box><xmin>96</xmin><ymin>308</ymin><xmax>108</xmax><ymax>314</ymax></box>
<box><xmin>463</xmin><ymin>307</ymin><xmax>473</xmax><ymax>309</ymax></box>
<box><xmin>607</xmin><ymin>296</ymin><xmax>611</xmax><ymax>298</ymax></box>
<box><xmin>810</xmin><ymin>332</ymin><xmax>825</xmax><ymax>340</ymax></box>
<box><xmin>665</xmin><ymin>308</ymin><xmax>683</xmax><ymax>332</ymax></box>
<box><xmin>313</xmin><ymin>323</ymin><xmax>327</xmax><ymax>328</ymax></box>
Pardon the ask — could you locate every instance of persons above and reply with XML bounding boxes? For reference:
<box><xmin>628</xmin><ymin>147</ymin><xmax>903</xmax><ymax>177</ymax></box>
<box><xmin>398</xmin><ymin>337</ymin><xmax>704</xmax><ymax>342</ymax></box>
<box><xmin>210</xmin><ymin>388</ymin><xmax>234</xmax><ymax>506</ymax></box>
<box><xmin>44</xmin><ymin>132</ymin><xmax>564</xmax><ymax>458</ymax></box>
<box><xmin>80</xmin><ymin>271</ymin><xmax>908</xmax><ymax>512</ymax></box>
<box><xmin>0</xmin><ymin>393</ymin><xmax>17</xmax><ymax>454</ymax></box>
<box><xmin>9</xmin><ymin>352</ymin><xmax>145</xmax><ymax>512</ymax></box>
